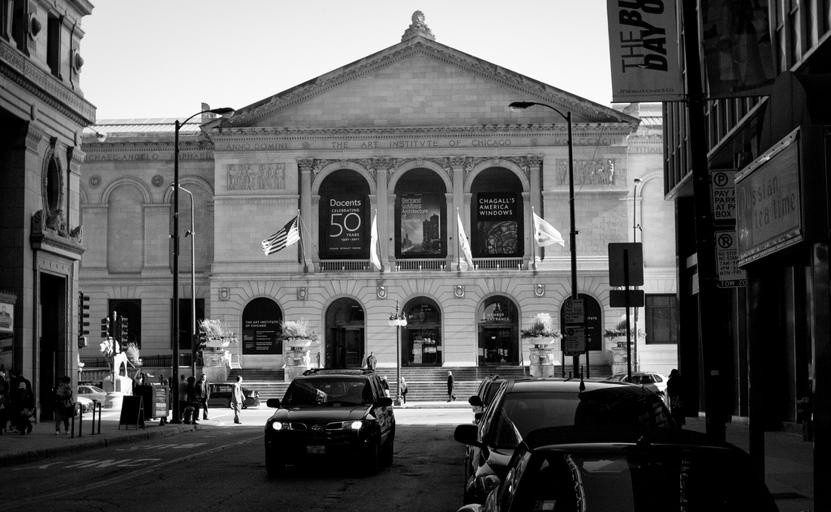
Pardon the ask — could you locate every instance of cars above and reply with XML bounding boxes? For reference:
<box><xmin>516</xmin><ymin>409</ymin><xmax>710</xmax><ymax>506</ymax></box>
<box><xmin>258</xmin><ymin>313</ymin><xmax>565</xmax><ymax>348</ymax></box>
<box><xmin>76</xmin><ymin>397</ymin><xmax>93</xmax><ymax>413</ymax></box>
<box><xmin>197</xmin><ymin>380</ymin><xmax>261</xmax><ymax>410</ymax></box>
<box><xmin>78</xmin><ymin>382</ymin><xmax>109</xmax><ymax>406</ymax></box>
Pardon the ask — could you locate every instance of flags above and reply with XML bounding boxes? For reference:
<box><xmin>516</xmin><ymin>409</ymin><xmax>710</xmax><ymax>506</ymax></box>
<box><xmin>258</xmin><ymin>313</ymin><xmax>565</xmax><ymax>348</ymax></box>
<box><xmin>455</xmin><ymin>214</ymin><xmax>473</xmax><ymax>266</ymax></box>
<box><xmin>369</xmin><ymin>216</ymin><xmax>379</xmax><ymax>268</ymax></box>
<box><xmin>261</xmin><ymin>215</ymin><xmax>299</xmax><ymax>256</ymax></box>
<box><xmin>535</xmin><ymin>214</ymin><xmax>564</xmax><ymax>247</ymax></box>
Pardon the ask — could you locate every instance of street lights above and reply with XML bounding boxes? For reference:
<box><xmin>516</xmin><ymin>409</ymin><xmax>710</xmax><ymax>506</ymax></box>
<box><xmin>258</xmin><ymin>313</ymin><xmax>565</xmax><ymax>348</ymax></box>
<box><xmin>171</xmin><ymin>104</ymin><xmax>239</xmax><ymax>425</ymax></box>
<box><xmin>632</xmin><ymin>177</ymin><xmax>643</xmax><ymax>372</ymax></box>
<box><xmin>508</xmin><ymin>97</ymin><xmax>581</xmax><ymax>377</ymax></box>
<box><xmin>170</xmin><ymin>181</ymin><xmax>200</xmax><ymax>381</ymax></box>
<box><xmin>388</xmin><ymin>299</ymin><xmax>410</xmax><ymax>406</ymax></box>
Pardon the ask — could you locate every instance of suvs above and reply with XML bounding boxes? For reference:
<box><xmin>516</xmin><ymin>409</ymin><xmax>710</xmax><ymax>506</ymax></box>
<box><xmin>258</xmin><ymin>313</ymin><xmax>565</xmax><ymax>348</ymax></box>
<box><xmin>465</xmin><ymin>367</ymin><xmax>669</xmax><ymax>499</ymax></box>
<box><xmin>262</xmin><ymin>364</ymin><xmax>398</xmax><ymax>473</ymax></box>
<box><xmin>454</xmin><ymin>422</ymin><xmax>782</xmax><ymax>511</ymax></box>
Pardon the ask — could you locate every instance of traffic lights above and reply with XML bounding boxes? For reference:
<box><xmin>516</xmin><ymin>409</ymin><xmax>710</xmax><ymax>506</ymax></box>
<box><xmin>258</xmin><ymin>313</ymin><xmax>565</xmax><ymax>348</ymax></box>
<box><xmin>100</xmin><ymin>316</ymin><xmax>111</xmax><ymax>340</ymax></box>
<box><xmin>119</xmin><ymin>313</ymin><xmax>129</xmax><ymax>354</ymax></box>
<box><xmin>193</xmin><ymin>331</ymin><xmax>206</xmax><ymax>355</ymax></box>
<box><xmin>79</xmin><ymin>290</ymin><xmax>92</xmax><ymax>338</ymax></box>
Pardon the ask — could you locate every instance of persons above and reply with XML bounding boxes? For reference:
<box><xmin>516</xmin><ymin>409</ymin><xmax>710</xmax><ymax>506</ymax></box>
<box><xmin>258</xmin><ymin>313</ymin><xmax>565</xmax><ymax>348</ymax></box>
<box><xmin>446</xmin><ymin>370</ymin><xmax>456</xmax><ymax>404</ymax></box>
<box><xmin>231</xmin><ymin>375</ymin><xmax>247</xmax><ymax>425</ymax></box>
<box><xmin>381</xmin><ymin>376</ymin><xmax>390</xmax><ymax>401</ymax></box>
<box><xmin>666</xmin><ymin>368</ymin><xmax>684</xmax><ymax>425</ymax></box>
<box><xmin>52</xmin><ymin>376</ymin><xmax>73</xmax><ymax>436</ymax></box>
<box><xmin>400</xmin><ymin>377</ymin><xmax>408</xmax><ymax>403</ymax></box>
<box><xmin>0</xmin><ymin>362</ymin><xmax>38</xmax><ymax>437</ymax></box>
<box><xmin>366</xmin><ymin>352</ymin><xmax>376</xmax><ymax>367</ymax></box>
<box><xmin>153</xmin><ymin>368</ymin><xmax>210</xmax><ymax>427</ymax></box>
